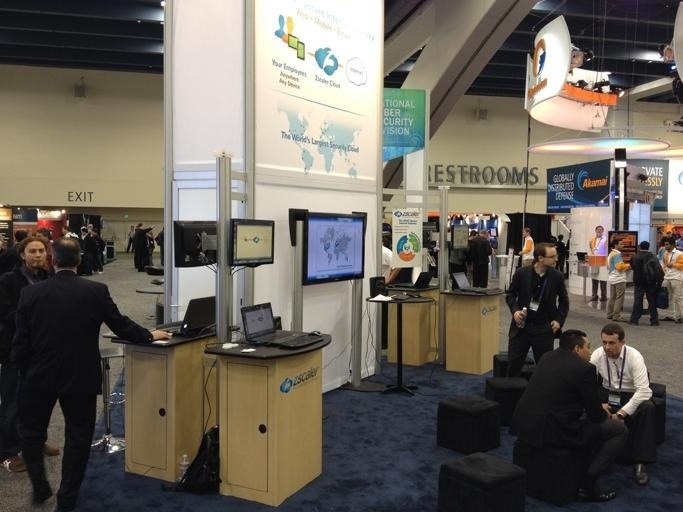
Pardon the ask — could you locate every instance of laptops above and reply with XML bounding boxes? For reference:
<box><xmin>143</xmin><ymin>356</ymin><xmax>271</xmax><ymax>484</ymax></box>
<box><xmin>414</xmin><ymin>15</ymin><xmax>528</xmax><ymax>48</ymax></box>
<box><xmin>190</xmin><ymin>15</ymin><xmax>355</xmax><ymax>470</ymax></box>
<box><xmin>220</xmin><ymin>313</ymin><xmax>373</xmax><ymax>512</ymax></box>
<box><xmin>397</xmin><ymin>272</ymin><xmax>432</xmax><ymax>288</ymax></box>
<box><xmin>451</xmin><ymin>271</ymin><xmax>488</xmax><ymax>292</ymax></box>
<box><xmin>241</xmin><ymin>303</ymin><xmax>306</xmax><ymax>344</ymax></box>
<box><xmin>158</xmin><ymin>296</ymin><xmax>215</xmax><ymax>336</ymax></box>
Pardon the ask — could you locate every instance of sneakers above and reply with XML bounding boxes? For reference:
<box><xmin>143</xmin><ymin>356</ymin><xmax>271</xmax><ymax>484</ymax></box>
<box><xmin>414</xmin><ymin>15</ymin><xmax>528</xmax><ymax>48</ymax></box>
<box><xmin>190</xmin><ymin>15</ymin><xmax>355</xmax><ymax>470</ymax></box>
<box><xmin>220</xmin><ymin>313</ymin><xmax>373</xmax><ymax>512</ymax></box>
<box><xmin>0</xmin><ymin>455</ymin><xmax>26</xmax><ymax>472</ymax></box>
<box><xmin>43</xmin><ymin>442</ymin><xmax>60</xmax><ymax>456</ymax></box>
<box><xmin>588</xmin><ymin>295</ymin><xmax>683</xmax><ymax>326</ymax></box>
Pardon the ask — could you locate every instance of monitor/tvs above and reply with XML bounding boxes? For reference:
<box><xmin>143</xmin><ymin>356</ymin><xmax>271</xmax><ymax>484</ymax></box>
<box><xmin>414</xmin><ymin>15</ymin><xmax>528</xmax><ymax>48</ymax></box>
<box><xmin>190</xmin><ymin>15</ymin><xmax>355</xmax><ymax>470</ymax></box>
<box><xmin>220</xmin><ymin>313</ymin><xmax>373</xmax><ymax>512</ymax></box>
<box><xmin>302</xmin><ymin>211</ymin><xmax>364</xmax><ymax>284</ymax></box>
<box><xmin>231</xmin><ymin>218</ymin><xmax>274</xmax><ymax>265</ymax></box>
<box><xmin>451</xmin><ymin>225</ymin><xmax>468</xmax><ymax>250</ymax></box>
<box><xmin>174</xmin><ymin>221</ymin><xmax>217</xmax><ymax>266</ymax></box>
<box><xmin>490</xmin><ymin>227</ymin><xmax>498</xmax><ymax>236</ymax></box>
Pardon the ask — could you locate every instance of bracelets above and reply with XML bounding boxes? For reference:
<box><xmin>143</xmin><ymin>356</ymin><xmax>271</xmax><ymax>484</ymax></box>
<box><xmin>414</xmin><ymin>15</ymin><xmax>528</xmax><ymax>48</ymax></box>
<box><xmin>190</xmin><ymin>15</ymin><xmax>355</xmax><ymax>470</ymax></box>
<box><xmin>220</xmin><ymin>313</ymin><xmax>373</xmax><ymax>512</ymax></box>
<box><xmin>615</xmin><ymin>411</ymin><xmax>625</xmax><ymax>418</ymax></box>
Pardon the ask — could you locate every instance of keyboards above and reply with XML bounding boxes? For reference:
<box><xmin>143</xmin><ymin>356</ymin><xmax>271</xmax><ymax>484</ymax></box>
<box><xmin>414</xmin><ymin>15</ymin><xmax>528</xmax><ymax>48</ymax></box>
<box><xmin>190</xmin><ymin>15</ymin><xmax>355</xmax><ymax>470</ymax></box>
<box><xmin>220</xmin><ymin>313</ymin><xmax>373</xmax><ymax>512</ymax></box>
<box><xmin>157</xmin><ymin>321</ymin><xmax>183</xmax><ymax>330</ymax></box>
<box><xmin>478</xmin><ymin>288</ymin><xmax>502</xmax><ymax>293</ymax></box>
<box><xmin>391</xmin><ymin>295</ymin><xmax>410</xmax><ymax>300</ymax></box>
<box><xmin>281</xmin><ymin>335</ymin><xmax>323</xmax><ymax>348</ymax></box>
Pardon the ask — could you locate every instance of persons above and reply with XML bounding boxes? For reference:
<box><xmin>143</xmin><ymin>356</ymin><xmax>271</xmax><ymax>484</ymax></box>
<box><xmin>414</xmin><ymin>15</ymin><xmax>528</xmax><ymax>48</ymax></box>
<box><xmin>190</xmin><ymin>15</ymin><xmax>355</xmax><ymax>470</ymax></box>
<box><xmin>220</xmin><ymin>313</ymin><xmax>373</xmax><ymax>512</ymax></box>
<box><xmin>382</xmin><ymin>246</ymin><xmax>401</xmax><ymax>283</ymax></box>
<box><xmin>588</xmin><ymin>226</ymin><xmax>683</xmax><ymax>326</ymax></box>
<box><xmin>0</xmin><ymin>230</ymin><xmax>62</xmax><ymax>476</ymax></box>
<box><xmin>508</xmin><ymin>325</ymin><xmax>620</xmax><ymax>504</ymax></box>
<box><xmin>501</xmin><ymin>240</ymin><xmax>572</xmax><ymax>379</ymax></box>
<box><xmin>520</xmin><ymin>228</ymin><xmax>535</xmax><ymax>267</ymax></box>
<box><xmin>8</xmin><ymin>235</ymin><xmax>173</xmax><ymax>511</ymax></box>
<box><xmin>586</xmin><ymin>318</ymin><xmax>660</xmax><ymax>487</ymax></box>
<box><xmin>0</xmin><ymin>212</ymin><xmax>165</xmax><ymax>278</ymax></box>
<box><xmin>555</xmin><ymin>234</ymin><xmax>567</xmax><ymax>272</ymax></box>
<box><xmin>448</xmin><ymin>230</ymin><xmax>494</xmax><ymax>289</ymax></box>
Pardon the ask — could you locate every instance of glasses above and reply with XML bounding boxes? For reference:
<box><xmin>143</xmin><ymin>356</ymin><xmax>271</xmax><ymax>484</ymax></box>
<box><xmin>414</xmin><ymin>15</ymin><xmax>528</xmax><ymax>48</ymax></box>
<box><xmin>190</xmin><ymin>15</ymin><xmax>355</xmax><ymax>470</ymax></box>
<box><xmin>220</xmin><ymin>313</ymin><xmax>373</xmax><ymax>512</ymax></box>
<box><xmin>544</xmin><ymin>254</ymin><xmax>558</xmax><ymax>259</ymax></box>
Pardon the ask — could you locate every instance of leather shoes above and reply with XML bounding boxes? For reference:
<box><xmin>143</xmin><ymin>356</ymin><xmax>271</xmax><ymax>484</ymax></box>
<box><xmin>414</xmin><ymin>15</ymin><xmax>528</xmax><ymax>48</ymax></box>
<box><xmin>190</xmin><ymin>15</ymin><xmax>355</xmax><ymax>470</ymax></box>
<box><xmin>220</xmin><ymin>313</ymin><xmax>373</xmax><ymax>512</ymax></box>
<box><xmin>575</xmin><ymin>486</ymin><xmax>616</xmax><ymax>503</ymax></box>
<box><xmin>633</xmin><ymin>463</ymin><xmax>648</xmax><ymax>485</ymax></box>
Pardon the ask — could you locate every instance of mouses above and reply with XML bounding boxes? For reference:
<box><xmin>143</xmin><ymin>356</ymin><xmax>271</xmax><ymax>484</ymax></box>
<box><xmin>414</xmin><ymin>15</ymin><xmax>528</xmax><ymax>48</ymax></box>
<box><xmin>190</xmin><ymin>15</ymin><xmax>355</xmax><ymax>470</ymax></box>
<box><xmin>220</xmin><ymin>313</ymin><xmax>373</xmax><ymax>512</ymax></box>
<box><xmin>388</xmin><ymin>284</ymin><xmax>393</xmax><ymax>288</ymax></box>
<box><xmin>413</xmin><ymin>295</ymin><xmax>418</xmax><ymax>298</ymax></box>
<box><xmin>308</xmin><ymin>331</ymin><xmax>323</xmax><ymax>336</ymax></box>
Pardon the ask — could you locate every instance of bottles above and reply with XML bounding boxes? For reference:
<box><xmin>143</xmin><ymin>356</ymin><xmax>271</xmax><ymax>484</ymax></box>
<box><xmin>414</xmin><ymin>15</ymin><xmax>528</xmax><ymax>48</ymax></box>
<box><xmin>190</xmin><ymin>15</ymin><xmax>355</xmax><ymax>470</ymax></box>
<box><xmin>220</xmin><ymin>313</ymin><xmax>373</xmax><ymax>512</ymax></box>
<box><xmin>177</xmin><ymin>453</ymin><xmax>190</xmax><ymax>481</ymax></box>
<box><xmin>517</xmin><ymin>306</ymin><xmax>527</xmax><ymax>329</ymax></box>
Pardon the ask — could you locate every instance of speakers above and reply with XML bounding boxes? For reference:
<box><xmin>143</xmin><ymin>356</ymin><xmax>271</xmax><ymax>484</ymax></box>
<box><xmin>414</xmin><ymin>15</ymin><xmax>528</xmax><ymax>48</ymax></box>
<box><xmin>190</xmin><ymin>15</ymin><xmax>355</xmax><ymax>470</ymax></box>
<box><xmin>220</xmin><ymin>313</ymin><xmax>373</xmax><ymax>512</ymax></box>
<box><xmin>370</xmin><ymin>277</ymin><xmax>385</xmax><ymax>297</ymax></box>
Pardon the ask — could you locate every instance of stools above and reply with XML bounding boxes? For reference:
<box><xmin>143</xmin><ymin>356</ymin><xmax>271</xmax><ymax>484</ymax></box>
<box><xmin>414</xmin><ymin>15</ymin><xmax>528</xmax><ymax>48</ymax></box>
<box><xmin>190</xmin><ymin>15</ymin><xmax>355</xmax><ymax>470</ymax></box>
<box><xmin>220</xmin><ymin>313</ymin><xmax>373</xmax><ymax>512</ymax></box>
<box><xmin>436</xmin><ymin>354</ymin><xmax>667</xmax><ymax>511</ymax></box>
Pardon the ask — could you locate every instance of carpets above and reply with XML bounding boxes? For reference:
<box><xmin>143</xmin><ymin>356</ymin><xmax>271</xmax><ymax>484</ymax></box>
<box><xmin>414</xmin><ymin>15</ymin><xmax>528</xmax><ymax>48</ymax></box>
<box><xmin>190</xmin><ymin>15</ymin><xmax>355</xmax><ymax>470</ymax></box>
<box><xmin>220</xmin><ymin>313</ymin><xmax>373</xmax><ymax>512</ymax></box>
<box><xmin>70</xmin><ymin>307</ymin><xmax>683</xmax><ymax>511</ymax></box>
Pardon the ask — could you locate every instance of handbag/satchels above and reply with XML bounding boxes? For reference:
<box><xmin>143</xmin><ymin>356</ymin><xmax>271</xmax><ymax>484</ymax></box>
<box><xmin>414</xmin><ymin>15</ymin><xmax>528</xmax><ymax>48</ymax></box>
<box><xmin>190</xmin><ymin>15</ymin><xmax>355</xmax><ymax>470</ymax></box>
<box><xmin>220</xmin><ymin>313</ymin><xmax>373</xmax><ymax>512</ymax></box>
<box><xmin>654</xmin><ymin>284</ymin><xmax>668</xmax><ymax>309</ymax></box>
